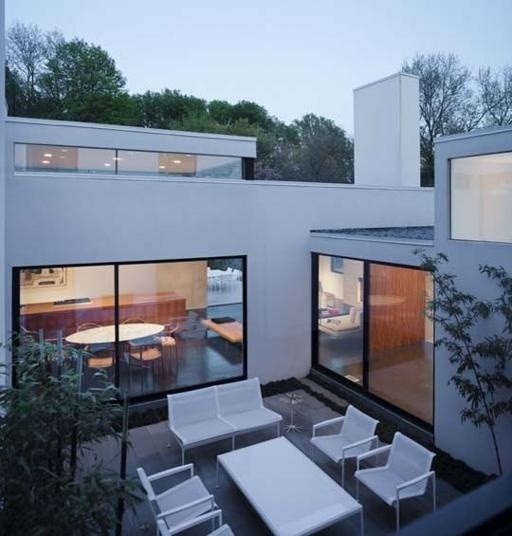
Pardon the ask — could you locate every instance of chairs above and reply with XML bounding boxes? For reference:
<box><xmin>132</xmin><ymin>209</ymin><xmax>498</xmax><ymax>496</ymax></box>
<box><xmin>77</xmin><ymin>317</ymin><xmax>177</xmax><ymax>396</ymax></box>
<box><xmin>138</xmin><ymin>464</ymin><xmax>235</xmax><ymax>536</ymax></box>
<box><xmin>309</xmin><ymin>404</ymin><xmax>438</xmax><ymax>535</ymax></box>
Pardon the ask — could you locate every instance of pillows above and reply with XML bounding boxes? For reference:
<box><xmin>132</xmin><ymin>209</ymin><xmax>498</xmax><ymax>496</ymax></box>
<box><xmin>212</xmin><ymin>316</ymin><xmax>235</xmax><ymax>324</ymax></box>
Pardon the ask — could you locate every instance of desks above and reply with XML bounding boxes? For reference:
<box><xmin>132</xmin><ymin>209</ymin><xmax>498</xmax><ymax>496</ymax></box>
<box><xmin>215</xmin><ymin>436</ymin><xmax>364</xmax><ymax>536</ymax></box>
<box><xmin>62</xmin><ymin>324</ymin><xmax>163</xmax><ymax>364</ymax></box>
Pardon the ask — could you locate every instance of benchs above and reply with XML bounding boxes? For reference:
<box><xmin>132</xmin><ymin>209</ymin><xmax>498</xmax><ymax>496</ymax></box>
<box><xmin>163</xmin><ymin>377</ymin><xmax>283</xmax><ymax>462</ymax></box>
<box><xmin>200</xmin><ymin>317</ymin><xmax>243</xmax><ymax>352</ymax></box>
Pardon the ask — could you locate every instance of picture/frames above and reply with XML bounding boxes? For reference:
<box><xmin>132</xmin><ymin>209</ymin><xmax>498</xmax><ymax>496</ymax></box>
<box><xmin>331</xmin><ymin>257</ymin><xmax>344</xmax><ymax>274</ymax></box>
<box><xmin>20</xmin><ymin>267</ymin><xmax>66</xmax><ymax>288</ymax></box>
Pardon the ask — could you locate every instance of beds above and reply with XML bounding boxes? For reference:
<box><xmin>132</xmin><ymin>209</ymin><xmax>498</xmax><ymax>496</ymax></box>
<box><xmin>318</xmin><ymin>313</ymin><xmax>361</xmax><ymax>338</ymax></box>
